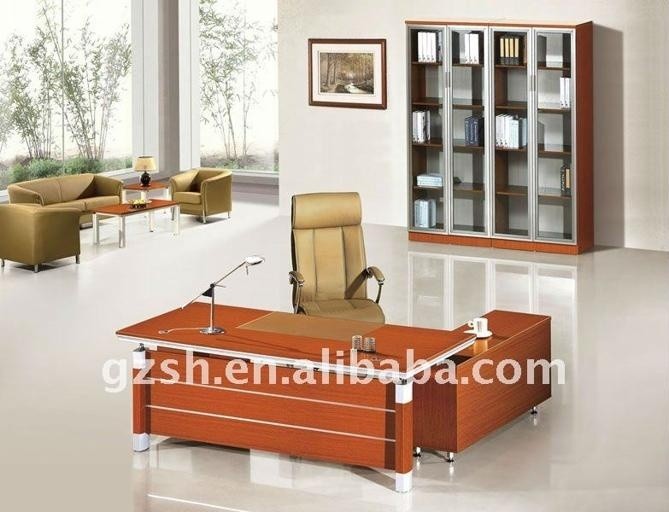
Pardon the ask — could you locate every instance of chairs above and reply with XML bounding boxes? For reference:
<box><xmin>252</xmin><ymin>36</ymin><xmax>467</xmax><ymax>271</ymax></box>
<box><xmin>288</xmin><ymin>191</ymin><xmax>386</xmax><ymax>323</ymax></box>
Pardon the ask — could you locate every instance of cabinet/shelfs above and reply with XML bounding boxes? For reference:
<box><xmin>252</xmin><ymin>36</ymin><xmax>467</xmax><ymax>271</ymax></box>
<box><xmin>404</xmin><ymin>17</ymin><xmax>594</xmax><ymax>256</ymax></box>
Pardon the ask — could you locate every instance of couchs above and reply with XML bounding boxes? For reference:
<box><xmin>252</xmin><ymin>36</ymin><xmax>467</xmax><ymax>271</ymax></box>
<box><xmin>169</xmin><ymin>167</ymin><xmax>232</xmax><ymax>223</ymax></box>
<box><xmin>0</xmin><ymin>202</ymin><xmax>82</xmax><ymax>274</ymax></box>
<box><xmin>7</xmin><ymin>173</ymin><xmax>123</xmax><ymax>230</ymax></box>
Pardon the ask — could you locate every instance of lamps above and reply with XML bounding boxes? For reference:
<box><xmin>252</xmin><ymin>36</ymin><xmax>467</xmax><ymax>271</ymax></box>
<box><xmin>134</xmin><ymin>156</ymin><xmax>156</xmax><ymax>187</ymax></box>
<box><xmin>180</xmin><ymin>255</ymin><xmax>265</xmax><ymax>333</ymax></box>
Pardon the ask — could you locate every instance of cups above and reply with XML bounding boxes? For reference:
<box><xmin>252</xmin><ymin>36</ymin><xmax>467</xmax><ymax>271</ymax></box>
<box><xmin>467</xmin><ymin>318</ymin><xmax>488</xmax><ymax>336</ymax></box>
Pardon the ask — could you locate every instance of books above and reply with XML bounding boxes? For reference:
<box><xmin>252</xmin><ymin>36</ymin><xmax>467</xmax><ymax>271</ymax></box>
<box><xmin>559</xmin><ymin>77</ymin><xmax>571</xmax><ymax>109</ymax></box>
<box><xmin>500</xmin><ymin>34</ymin><xmax>528</xmax><ymax>65</ymax></box>
<box><xmin>414</xmin><ymin>194</ymin><xmax>438</xmax><ymax>228</ymax></box>
<box><xmin>464</xmin><ymin>32</ymin><xmax>481</xmax><ymax>64</ymax></box>
<box><xmin>463</xmin><ymin>114</ymin><xmax>484</xmax><ymax>148</ymax></box>
<box><xmin>417</xmin><ymin>31</ymin><xmax>442</xmax><ymax>62</ymax></box>
<box><xmin>496</xmin><ymin>112</ymin><xmax>527</xmax><ymax>149</ymax></box>
<box><xmin>412</xmin><ymin>108</ymin><xmax>437</xmax><ymax>143</ymax></box>
<box><xmin>560</xmin><ymin>164</ymin><xmax>572</xmax><ymax>197</ymax></box>
<box><xmin>416</xmin><ymin>172</ymin><xmax>444</xmax><ymax>187</ymax></box>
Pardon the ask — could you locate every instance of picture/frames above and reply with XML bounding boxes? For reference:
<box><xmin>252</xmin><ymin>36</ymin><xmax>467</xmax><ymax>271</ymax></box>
<box><xmin>308</xmin><ymin>37</ymin><xmax>387</xmax><ymax>110</ymax></box>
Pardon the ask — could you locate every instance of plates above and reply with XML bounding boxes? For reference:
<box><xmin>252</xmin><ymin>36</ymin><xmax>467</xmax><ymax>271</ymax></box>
<box><xmin>125</xmin><ymin>200</ymin><xmax>152</xmax><ymax>209</ymax></box>
<box><xmin>464</xmin><ymin>330</ymin><xmax>493</xmax><ymax>338</ymax></box>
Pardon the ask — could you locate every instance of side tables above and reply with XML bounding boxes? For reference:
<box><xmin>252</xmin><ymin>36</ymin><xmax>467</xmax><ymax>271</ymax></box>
<box><xmin>124</xmin><ymin>182</ymin><xmax>169</xmax><ymax>203</ymax></box>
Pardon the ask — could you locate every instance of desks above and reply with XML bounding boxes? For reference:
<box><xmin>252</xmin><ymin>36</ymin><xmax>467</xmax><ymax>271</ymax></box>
<box><xmin>115</xmin><ymin>301</ymin><xmax>551</xmax><ymax>494</ymax></box>
